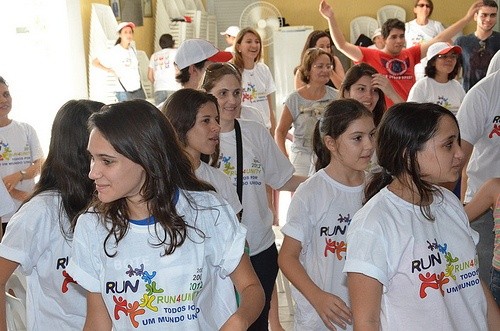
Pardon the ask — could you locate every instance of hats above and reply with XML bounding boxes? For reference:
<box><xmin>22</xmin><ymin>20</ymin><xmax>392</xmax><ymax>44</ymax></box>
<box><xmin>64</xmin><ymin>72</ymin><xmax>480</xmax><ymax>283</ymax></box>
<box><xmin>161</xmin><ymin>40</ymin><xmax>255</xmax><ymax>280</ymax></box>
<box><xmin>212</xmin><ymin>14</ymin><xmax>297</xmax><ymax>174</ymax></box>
<box><xmin>174</xmin><ymin>39</ymin><xmax>233</xmax><ymax>70</ymax></box>
<box><xmin>220</xmin><ymin>25</ymin><xmax>241</xmax><ymax>38</ymax></box>
<box><xmin>372</xmin><ymin>28</ymin><xmax>382</xmax><ymax>41</ymax></box>
<box><xmin>427</xmin><ymin>41</ymin><xmax>462</xmax><ymax>60</ymax></box>
<box><xmin>118</xmin><ymin>21</ymin><xmax>135</xmax><ymax>32</ymax></box>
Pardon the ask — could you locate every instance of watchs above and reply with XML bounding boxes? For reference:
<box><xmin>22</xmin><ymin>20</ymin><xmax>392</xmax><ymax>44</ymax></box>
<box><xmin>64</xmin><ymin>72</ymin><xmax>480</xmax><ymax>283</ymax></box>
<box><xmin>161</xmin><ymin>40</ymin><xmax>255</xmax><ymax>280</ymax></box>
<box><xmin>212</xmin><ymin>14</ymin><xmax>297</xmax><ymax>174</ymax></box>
<box><xmin>20</xmin><ymin>169</ymin><xmax>26</xmax><ymax>181</ymax></box>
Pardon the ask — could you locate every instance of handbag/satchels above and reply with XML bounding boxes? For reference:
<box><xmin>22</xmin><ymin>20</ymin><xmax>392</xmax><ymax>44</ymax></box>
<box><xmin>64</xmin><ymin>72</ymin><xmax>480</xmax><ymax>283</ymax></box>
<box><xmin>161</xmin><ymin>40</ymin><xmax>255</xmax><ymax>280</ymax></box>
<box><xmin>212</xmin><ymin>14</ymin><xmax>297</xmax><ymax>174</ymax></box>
<box><xmin>126</xmin><ymin>88</ymin><xmax>147</xmax><ymax>101</ymax></box>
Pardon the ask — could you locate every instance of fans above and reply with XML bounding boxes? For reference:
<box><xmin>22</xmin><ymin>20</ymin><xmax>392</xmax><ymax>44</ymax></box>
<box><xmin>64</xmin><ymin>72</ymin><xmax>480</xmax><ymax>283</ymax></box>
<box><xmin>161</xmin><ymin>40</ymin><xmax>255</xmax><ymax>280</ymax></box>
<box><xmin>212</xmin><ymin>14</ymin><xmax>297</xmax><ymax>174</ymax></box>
<box><xmin>239</xmin><ymin>1</ymin><xmax>282</xmax><ymax>60</ymax></box>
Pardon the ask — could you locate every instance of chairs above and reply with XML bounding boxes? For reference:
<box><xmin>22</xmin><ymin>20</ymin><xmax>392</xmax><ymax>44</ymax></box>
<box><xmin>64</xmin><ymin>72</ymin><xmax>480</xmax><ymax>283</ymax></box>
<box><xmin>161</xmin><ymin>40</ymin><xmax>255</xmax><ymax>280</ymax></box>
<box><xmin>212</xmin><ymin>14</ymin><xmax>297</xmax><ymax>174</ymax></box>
<box><xmin>88</xmin><ymin>4</ymin><xmax>152</xmax><ymax>101</ymax></box>
<box><xmin>155</xmin><ymin>0</ymin><xmax>217</xmax><ymax>56</ymax></box>
<box><xmin>350</xmin><ymin>5</ymin><xmax>406</xmax><ymax>44</ymax></box>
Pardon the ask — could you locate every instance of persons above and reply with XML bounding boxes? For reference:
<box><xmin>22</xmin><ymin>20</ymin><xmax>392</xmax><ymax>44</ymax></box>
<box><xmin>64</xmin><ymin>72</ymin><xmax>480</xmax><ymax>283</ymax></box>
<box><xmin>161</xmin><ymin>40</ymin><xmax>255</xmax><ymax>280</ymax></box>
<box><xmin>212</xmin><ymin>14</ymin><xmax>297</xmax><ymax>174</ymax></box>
<box><xmin>219</xmin><ymin>28</ymin><xmax>240</xmax><ymax>54</ymax></box>
<box><xmin>0</xmin><ymin>79</ymin><xmax>46</xmax><ymax>235</ymax></box>
<box><xmin>201</xmin><ymin>63</ymin><xmax>310</xmax><ymax>331</ymax></box>
<box><xmin>0</xmin><ymin>100</ymin><xmax>107</xmax><ymax>330</ymax></box>
<box><xmin>227</xmin><ymin>28</ymin><xmax>277</xmax><ymax>137</ymax></box>
<box><xmin>67</xmin><ymin>100</ymin><xmax>266</xmax><ymax>331</ymax></box>
<box><xmin>158</xmin><ymin>40</ymin><xmax>233</xmax><ymax>109</ymax></box>
<box><xmin>274</xmin><ymin>0</ymin><xmax>500</xmax><ymax>331</ymax></box>
<box><xmin>161</xmin><ymin>89</ymin><xmax>243</xmax><ymax>224</ymax></box>
<box><xmin>91</xmin><ymin>22</ymin><xmax>146</xmax><ymax>102</ymax></box>
<box><xmin>148</xmin><ymin>34</ymin><xmax>184</xmax><ymax>104</ymax></box>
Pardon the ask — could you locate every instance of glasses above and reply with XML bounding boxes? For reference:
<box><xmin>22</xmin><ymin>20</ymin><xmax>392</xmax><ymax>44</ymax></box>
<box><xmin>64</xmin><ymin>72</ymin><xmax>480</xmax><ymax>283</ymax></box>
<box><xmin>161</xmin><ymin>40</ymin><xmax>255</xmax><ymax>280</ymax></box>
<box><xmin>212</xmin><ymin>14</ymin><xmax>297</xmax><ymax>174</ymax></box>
<box><xmin>416</xmin><ymin>4</ymin><xmax>431</xmax><ymax>8</ymax></box>
<box><xmin>314</xmin><ymin>64</ymin><xmax>333</xmax><ymax>71</ymax></box>
<box><xmin>438</xmin><ymin>54</ymin><xmax>456</xmax><ymax>59</ymax></box>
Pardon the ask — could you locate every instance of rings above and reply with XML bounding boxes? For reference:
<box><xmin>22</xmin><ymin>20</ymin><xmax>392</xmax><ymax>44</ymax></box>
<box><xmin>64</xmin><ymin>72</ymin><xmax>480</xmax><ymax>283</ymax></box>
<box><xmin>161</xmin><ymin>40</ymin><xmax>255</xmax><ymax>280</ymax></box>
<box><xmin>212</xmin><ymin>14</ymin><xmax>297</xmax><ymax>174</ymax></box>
<box><xmin>8</xmin><ymin>183</ymin><xmax>10</xmax><ymax>186</ymax></box>
